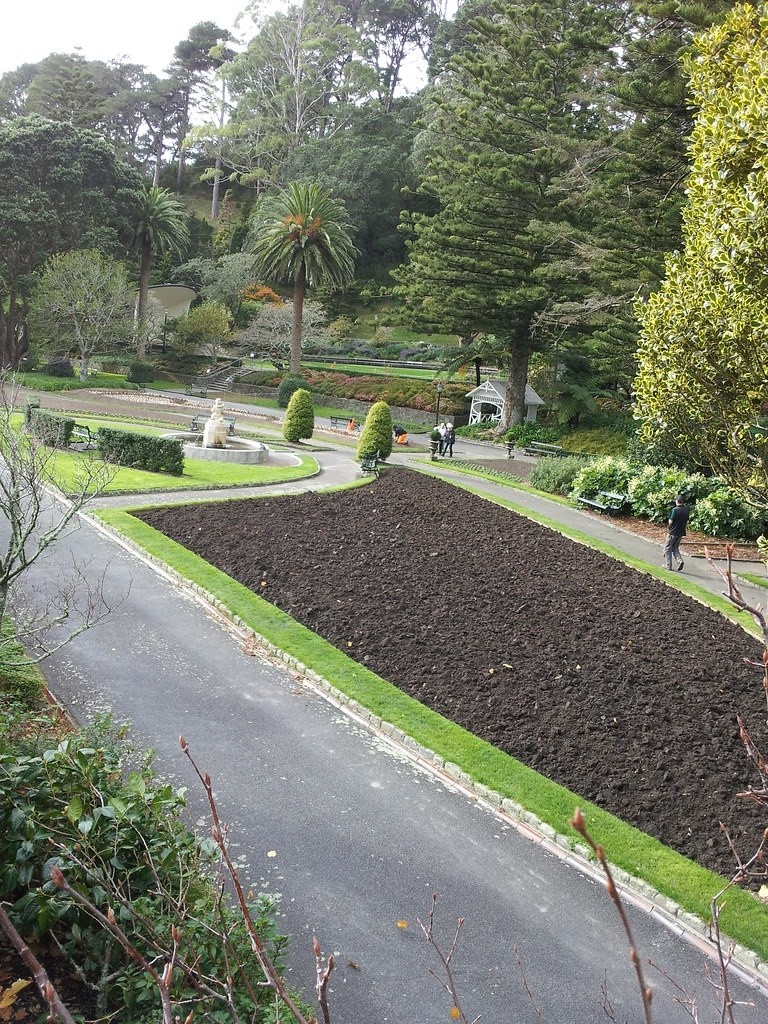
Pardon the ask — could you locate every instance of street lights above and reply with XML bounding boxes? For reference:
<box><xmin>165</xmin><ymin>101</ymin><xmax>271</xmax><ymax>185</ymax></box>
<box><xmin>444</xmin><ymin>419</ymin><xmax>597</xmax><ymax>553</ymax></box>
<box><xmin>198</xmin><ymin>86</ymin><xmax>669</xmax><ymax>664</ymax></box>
<box><xmin>163</xmin><ymin>307</ymin><xmax>168</xmax><ymax>354</ymax></box>
<box><xmin>434</xmin><ymin>381</ymin><xmax>443</xmax><ymax>427</ymax></box>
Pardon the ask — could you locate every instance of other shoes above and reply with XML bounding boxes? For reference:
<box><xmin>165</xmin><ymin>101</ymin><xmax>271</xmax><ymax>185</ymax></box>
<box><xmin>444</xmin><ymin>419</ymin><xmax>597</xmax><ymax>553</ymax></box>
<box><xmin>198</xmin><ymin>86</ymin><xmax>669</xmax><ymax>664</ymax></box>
<box><xmin>662</xmin><ymin>564</ymin><xmax>671</xmax><ymax>570</ymax></box>
<box><xmin>677</xmin><ymin>561</ymin><xmax>684</xmax><ymax>570</ymax></box>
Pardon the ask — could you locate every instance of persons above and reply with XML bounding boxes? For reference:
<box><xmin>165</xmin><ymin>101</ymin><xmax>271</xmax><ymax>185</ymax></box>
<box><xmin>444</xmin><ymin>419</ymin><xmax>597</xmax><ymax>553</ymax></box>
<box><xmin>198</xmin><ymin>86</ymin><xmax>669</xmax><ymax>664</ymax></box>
<box><xmin>434</xmin><ymin>422</ymin><xmax>455</xmax><ymax>457</ymax></box>
<box><xmin>661</xmin><ymin>495</ymin><xmax>689</xmax><ymax>571</ymax></box>
<box><xmin>211</xmin><ymin>398</ymin><xmax>224</xmax><ymax>422</ymax></box>
<box><xmin>347</xmin><ymin>415</ymin><xmax>355</xmax><ymax>431</ymax></box>
<box><xmin>393</xmin><ymin>426</ymin><xmax>408</xmax><ymax>445</ymax></box>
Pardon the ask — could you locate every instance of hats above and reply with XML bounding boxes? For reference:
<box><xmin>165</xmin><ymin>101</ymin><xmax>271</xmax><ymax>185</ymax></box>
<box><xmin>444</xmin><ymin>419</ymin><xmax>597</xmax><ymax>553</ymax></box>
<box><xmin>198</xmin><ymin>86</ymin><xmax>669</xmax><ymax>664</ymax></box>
<box><xmin>674</xmin><ymin>495</ymin><xmax>684</xmax><ymax>502</ymax></box>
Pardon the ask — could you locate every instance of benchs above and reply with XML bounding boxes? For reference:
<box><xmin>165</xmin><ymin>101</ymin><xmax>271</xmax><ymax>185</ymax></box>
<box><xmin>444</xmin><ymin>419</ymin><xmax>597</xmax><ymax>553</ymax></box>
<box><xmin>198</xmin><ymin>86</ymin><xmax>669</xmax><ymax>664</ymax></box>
<box><xmin>86</xmin><ymin>367</ymin><xmax>98</xmax><ymax>378</ymax></box>
<box><xmin>524</xmin><ymin>441</ymin><xmax>563</xmax><ymax>459</ymax></box>
<box><xmin>577</xmin><ymin>490</ymin><xmax>627</xmax><ymax>515</ymax></box>
<box><xmin>360</xmin><ymin>449</ymin><xmax>381</xmax><ymax>479</ymax></box>
<box><xmin>185</xmin><ymin>385</ymin><xmax>208</xmax><ymax>398</ymax></box>
<box><xmin>330</xmin><ymin>416</ymin><xmax>361</xmax><ymax>432</ymax></box>
<box><xmin>190</xmin><ymin>411</ymin><xmax>238</xmax><ymax>436</ymax></box>
<box><xmin>69</xmin><ymin>423</ymin><xmax>100</xmax><ymax>450</ymax></box>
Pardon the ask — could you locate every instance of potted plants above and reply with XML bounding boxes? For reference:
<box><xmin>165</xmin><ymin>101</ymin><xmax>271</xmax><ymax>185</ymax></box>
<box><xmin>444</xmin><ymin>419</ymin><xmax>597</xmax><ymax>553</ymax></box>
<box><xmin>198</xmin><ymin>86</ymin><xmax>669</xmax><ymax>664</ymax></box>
<box><xmin>504</xmin><ymin>432</ymin><xmax>517</xmax><ymax>455</ymax></box>
<box><xmin>428</xmin><ymin>430</ymin><xmax>442</xmax><ymax>448</ymax></box>
